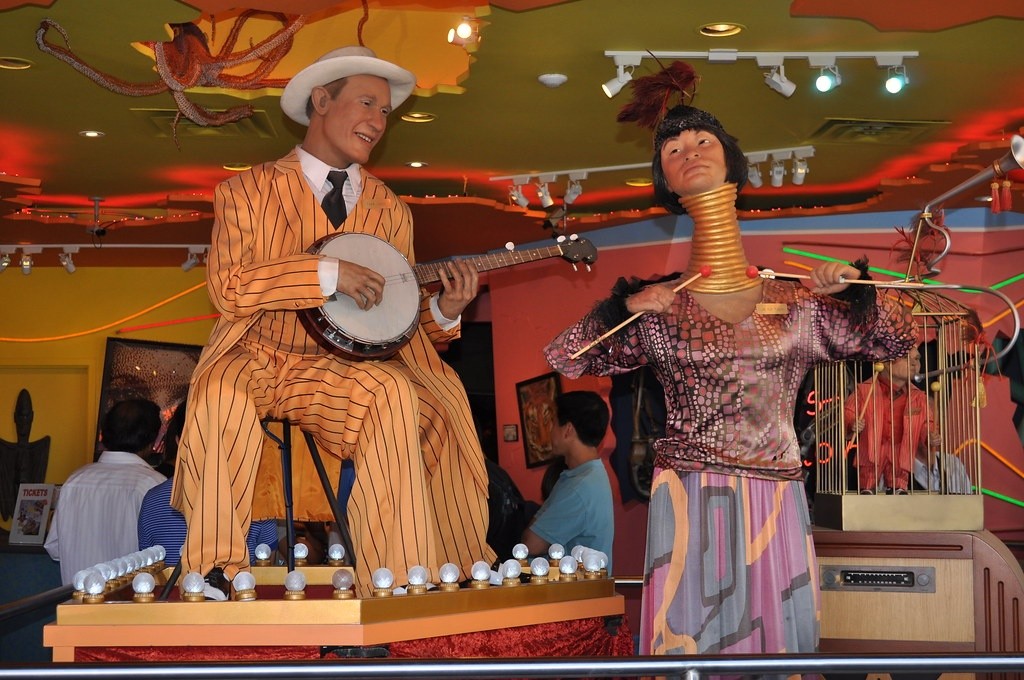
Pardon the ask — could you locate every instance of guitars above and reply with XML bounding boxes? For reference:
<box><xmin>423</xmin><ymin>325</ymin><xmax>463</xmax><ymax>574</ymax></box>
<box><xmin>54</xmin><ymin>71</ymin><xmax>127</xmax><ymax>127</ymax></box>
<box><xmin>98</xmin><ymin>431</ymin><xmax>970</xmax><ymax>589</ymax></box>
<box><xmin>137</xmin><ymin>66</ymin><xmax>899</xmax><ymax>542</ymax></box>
<box><xmin>293</xmin><ymin>227</ymin><xmax>597</xmax><ymax>363</ymax></box>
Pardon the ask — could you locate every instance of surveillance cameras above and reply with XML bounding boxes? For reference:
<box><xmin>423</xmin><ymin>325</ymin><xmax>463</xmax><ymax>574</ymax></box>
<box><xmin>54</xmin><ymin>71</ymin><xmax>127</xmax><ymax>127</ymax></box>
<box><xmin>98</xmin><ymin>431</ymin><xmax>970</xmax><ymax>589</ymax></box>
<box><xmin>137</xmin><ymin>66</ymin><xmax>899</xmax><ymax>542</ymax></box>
<box><xmin>86</xmin><ymin>226</ymin><xmax>106</xmax><ymax>236</ymax></box>
<box><xmin>542</xmin><ymin>207</ymin><xmax>565</xmax><ymax>229</ymax></box>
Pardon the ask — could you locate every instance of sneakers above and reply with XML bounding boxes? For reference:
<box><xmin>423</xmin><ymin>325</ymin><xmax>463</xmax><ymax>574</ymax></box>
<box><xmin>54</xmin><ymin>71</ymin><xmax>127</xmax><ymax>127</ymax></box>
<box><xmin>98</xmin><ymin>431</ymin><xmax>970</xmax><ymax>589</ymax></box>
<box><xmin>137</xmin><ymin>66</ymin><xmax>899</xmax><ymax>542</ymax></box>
<box><xmin>393</xmin><ymin>583</ymin><xmax>440</xmax><ymax>596</ymax></box>
<box><xmin>203</xmin><ymin>567</ymin><xmax>230</xmax><ymax>600</ymax></box>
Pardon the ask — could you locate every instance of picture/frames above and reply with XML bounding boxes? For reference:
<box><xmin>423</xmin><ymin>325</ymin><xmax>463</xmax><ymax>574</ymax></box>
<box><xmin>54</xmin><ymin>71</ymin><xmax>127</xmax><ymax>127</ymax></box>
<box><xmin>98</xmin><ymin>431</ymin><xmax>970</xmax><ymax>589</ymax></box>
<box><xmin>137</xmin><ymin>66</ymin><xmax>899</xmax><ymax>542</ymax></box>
<box><xmin>96</xmin><ymin>337</ymin><xmax>204</xmax><ymax>471</ymax></box>
<box><xmin>514</xmin><ymin>372</ymin><xmax>564</xmax><ymax>466</ymax></box>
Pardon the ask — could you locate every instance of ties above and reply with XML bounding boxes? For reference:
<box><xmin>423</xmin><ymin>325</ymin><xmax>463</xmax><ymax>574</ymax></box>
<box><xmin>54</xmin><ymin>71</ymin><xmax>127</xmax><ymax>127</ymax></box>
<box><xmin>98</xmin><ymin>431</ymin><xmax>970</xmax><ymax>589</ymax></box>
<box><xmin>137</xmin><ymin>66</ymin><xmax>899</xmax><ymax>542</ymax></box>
<box><xmin>321</xmin><ymin>171</ymin><xmax>349</xmax><ymax>230</ymax></box>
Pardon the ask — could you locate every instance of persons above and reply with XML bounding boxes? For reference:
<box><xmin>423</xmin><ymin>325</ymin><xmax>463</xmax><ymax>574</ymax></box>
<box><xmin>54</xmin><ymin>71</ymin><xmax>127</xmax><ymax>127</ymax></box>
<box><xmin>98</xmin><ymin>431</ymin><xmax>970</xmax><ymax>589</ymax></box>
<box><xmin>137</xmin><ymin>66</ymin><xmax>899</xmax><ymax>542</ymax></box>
<box><xmin>844</xmin><ymin>346</ymin><xmax>941</xmax><ymax>494</ymax></box>
<box><xmin>541</xmin><ymin>103</ymin><xmax>918</xmax><ymax>680</ymax></box>
<box><xmin>523</xmin><ymin>390</ymin><xmax>615</xmax><ymax>576</ymax></box>
<box><xmin>138</xmin><ymin>400</ymin><xmax>279</xmax><ymax>569</ymax></box>
<box><xmin>41</xmin><ymin>397</ymin><xmax>170</xmax><ymax>585</ymax></box>
<box><xmin>169</xmin><ymin>45</ymin><xmax>499</xmax><ymax>601</ymax></box>
<box><xmin>877</xmin><ymin>449</ymin><xmax>971</xmax><ymax>494</ymax></box>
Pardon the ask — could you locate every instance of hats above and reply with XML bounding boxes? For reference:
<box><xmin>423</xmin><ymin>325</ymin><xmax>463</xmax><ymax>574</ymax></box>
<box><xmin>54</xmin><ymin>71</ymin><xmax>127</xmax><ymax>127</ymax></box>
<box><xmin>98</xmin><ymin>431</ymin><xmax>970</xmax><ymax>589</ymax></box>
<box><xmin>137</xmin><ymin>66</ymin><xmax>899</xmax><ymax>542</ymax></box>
<box><xmin>280</xmin><ymin>45</ymin><xmax>416</xmax><ymax>126</ymax></box>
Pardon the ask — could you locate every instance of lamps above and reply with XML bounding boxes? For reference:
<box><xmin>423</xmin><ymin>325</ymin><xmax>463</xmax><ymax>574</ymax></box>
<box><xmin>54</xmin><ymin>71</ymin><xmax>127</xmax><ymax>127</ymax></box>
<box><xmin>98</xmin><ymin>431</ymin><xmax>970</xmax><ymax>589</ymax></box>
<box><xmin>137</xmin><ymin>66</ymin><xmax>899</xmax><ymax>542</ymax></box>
<box><xmin>0</xmin><ymin>247</ymin><xmax>18</xmax><ymax>271</ymax></box>
<box><xmin>748</xmin><ymin>156</ymin><xmax>767</xmax><ymax>187</ymax></box>
<box><xmin>564</xmin><ymin>171</ymin><xmax>590</xmax><ymax>205</ymax></box>
<box><xmin>807</xmin><ymin>52</ymin><xmax>847</xmax><ymax>92</ymax></box>
<box><xmin>180</xmin><ymin>246</ymin><xmax>206</xmax><ymax>272</ymax></box>
<box><xmin>765</xmin><ymin>59</ymin><xmax>798</xmax><ymax>95</ymax></box>
<box><xmin>534</xmin><ymin>173</ymin><xmax>556</xmax><ymax>206</ymax></box>
<box><xmin>20</xmin><ymin>246</ymin><xmax>44</xmax><ymax>274</ymax></box>
<box><xmin>57</xmin><ymin>247</ymin><xmax>82</xmax><ymax>273</ymax></box>
<box><xmin>768</xmin><ymin>150</ymin><xmax>792</xmax><ymax>187</ymax></box>
<box><xmin>872</xmin><ymin>54</ymin><xmax>914</xmax><ymax>94</ymax></box>
<box><xmin>508</xmin><ymin>176</ymin><xmax>532</xmax><ymax>207</ymax></box>
<box><xmin>792</xmin><ymin>148</ymin><xmax>816</xmax><ymax>186</ymax></box>
<box><xmin>446</xmin><ymin>15</ymin><xmax>482</xmax><ymax>48</ymax></box>
<box><xmin>603</xmin><ymin>56</ymin><xmax>642</xmax><ymax>96</ymax></box>
